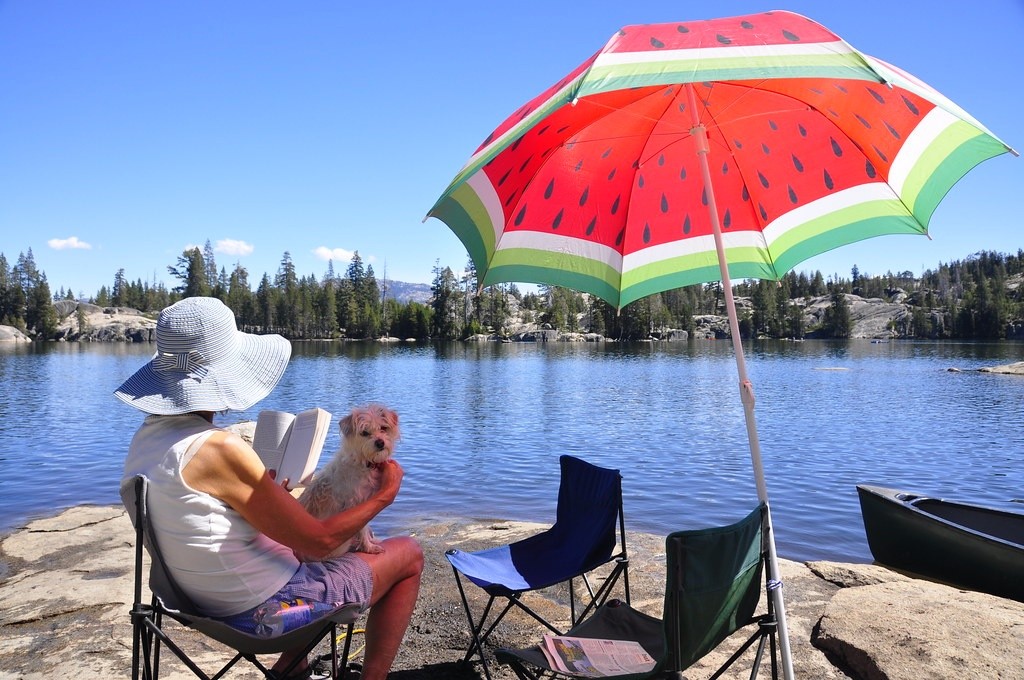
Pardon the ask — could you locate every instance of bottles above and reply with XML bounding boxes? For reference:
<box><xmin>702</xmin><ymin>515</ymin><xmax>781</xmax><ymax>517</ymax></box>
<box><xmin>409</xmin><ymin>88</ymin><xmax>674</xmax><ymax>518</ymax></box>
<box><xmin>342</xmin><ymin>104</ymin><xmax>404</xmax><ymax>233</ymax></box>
<box><xmin>253</xmin><ymin>597</ymin><xmax>343</xmax><ymax>638</ymax></box>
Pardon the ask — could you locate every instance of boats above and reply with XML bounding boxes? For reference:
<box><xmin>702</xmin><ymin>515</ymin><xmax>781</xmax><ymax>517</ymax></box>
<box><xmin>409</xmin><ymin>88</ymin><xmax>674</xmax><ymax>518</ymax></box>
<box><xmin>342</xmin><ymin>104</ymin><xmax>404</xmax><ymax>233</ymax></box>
<box><xmin>856</xmin><ymin>484</ymin><xmax>1024</xmax><ymax>602</ymax></box>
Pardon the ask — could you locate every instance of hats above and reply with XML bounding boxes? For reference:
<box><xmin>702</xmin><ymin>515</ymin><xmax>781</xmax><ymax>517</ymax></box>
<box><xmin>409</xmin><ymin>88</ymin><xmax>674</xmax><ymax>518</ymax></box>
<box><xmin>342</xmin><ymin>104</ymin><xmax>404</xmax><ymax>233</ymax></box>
<box><xmin>112</xmin><ymin>296</ymin><xmax>292</xmax><ymax>415</ymax></box>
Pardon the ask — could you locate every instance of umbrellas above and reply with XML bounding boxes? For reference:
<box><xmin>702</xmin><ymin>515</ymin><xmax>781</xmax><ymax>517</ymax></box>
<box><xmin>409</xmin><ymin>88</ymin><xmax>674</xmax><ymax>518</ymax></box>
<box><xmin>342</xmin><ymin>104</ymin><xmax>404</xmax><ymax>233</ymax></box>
<box><xmin>423</xmin><ymin>10</ymin><xmax>1019</xmax><ymax>680</ymax></box>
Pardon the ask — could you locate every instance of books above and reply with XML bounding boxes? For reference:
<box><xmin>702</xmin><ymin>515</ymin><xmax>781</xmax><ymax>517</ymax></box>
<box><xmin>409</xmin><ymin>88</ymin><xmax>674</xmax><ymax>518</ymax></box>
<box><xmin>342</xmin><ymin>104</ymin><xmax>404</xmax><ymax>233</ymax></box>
<box><xmin>252</xmin><ymin>408</ymin><xmax>332</xmax><ymax>488</ymax></box>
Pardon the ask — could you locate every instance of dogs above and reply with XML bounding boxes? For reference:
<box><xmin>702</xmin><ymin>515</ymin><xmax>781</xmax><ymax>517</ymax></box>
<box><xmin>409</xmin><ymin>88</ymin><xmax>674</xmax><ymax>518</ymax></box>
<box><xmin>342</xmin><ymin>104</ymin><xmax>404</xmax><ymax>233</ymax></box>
<box><xmin>296</xmin><ymin>403</ymin><xmax>401</xmax><ymax>555</ymax></box>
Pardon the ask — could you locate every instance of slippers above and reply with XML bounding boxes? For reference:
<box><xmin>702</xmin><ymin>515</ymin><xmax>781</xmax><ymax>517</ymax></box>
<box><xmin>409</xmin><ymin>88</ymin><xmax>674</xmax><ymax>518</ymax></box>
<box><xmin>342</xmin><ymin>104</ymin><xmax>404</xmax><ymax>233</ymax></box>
<box><xmin>267</xmin><ymin>662</ymin><xmax>311</xmax><ymax>680</ymax></box>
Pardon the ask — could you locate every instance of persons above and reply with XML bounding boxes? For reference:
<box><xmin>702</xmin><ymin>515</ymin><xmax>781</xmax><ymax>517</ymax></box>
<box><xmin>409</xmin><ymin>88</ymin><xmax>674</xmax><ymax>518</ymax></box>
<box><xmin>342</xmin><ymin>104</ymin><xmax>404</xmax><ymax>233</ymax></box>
<box><xmin>114</xmin><ymin>296</ymin><xmax>425</xmax><ymax>680</ymax></box>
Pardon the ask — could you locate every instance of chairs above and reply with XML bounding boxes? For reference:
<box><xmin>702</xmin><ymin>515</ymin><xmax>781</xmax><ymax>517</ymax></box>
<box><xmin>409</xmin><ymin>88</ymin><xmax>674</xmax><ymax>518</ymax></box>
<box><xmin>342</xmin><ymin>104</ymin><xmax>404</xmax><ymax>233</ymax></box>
<box><xmin>445</xmin><ymin>454</ymin><xmax>624</xmax><ymax>680</ymax></box>
<box><xmin>495</xmin><ymin>502</ymin><xmax>776</xmax><ymax>680</ymax></box>
<box><xmin>118</xmin><ymin>476</ymin><xmax>355</xmax><ymax>680</ymax></box>
